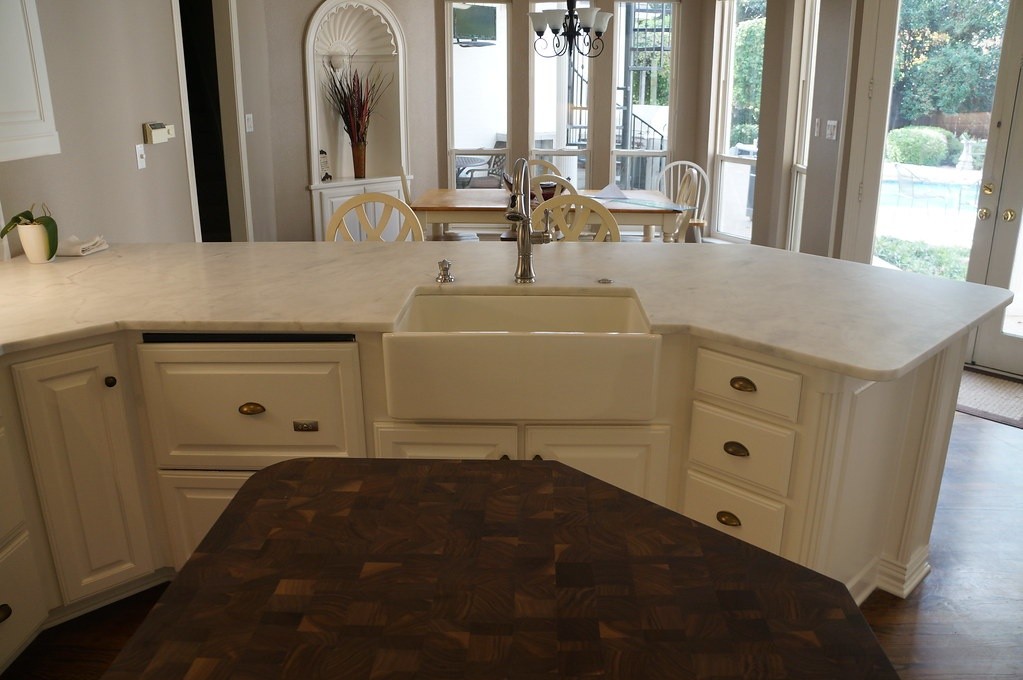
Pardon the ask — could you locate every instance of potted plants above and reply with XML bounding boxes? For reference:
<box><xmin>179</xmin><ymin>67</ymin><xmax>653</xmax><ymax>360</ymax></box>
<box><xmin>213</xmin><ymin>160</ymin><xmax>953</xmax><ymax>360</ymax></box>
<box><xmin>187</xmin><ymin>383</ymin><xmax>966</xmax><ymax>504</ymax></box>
<box><xmin>1</xmin><ymin>206</ymin><xmax>58</xmax><ymax>265</ymax></box>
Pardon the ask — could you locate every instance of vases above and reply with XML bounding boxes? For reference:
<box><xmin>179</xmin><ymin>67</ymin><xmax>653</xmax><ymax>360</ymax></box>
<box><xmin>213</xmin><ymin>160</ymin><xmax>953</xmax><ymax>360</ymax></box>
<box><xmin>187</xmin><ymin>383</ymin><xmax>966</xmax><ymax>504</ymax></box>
<box><xmin>351</xmin><ymin>141</ymin><xmax>366</xmax><ymax>180</ymax></box>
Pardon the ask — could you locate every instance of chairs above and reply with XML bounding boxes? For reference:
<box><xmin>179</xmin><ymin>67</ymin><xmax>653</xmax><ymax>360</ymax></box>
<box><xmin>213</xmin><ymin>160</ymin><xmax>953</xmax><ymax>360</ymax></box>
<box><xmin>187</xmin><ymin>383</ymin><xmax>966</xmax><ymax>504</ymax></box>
<box><xmin>325</xmin><ymin>160</ymin><xmax>709</xmax><ymax>243</ymax></box>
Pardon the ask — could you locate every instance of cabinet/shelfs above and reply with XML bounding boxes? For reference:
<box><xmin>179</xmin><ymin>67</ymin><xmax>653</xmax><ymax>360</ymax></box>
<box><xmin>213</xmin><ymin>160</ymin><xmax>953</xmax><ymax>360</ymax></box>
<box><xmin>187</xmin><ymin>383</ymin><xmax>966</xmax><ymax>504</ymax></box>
<box><xmin>0</xmin><ymin>282</ymin><xmax>942</xmax><ymax>680</ymax></box>
<box><xmin>305</xmin><ymin>1</ymin><xmax>413</xmax><ymax>243</ymax></box>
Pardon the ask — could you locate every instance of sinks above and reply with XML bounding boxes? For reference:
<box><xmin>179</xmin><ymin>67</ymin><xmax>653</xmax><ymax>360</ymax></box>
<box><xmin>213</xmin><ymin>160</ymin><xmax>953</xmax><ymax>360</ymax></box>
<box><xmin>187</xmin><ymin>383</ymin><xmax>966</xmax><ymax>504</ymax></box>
<box><xmin>381</xmin><ymin>286</ymin><xmax>663</xmax><ymax>421</ymax></box>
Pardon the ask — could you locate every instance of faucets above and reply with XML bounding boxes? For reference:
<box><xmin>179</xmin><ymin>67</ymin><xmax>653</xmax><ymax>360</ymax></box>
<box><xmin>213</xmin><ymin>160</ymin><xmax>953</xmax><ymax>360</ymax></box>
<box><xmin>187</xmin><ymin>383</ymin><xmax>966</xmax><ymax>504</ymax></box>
<box><xmin>504</xmin><ymin>158</ymin><xmax>552</xmax><ymax>285</ymax></box>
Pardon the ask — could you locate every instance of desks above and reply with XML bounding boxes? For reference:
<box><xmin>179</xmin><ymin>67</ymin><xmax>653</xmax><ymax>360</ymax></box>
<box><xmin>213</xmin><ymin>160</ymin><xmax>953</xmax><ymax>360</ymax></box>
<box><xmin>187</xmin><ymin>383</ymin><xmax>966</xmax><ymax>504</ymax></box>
<box><xmin>101</xmin><ymin>460</ymin><xmax>899</xmax><ymax>680</ymax></box>
<box><xmin>411</xmin><ymin>188</ymin><xmax>682</xmax><ymax>244</ymax></box>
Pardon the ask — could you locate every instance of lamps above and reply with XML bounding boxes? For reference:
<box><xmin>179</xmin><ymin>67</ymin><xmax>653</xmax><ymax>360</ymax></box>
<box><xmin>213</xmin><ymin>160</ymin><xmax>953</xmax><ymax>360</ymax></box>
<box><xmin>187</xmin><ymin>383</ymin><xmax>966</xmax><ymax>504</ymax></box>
<box><xmin>530</xmin><ymin>0</ymin><xmax>614</xmax><ymax>59</ymax></box>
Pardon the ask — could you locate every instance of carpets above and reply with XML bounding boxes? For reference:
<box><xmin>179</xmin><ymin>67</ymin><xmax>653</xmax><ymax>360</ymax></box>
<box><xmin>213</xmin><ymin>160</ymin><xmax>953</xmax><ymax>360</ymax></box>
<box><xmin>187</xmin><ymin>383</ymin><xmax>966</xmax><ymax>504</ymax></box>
<box><xmin>956</xmin><ymin>370</ymin><xmax>1023</xmax><ymax>430</ymax></box>
